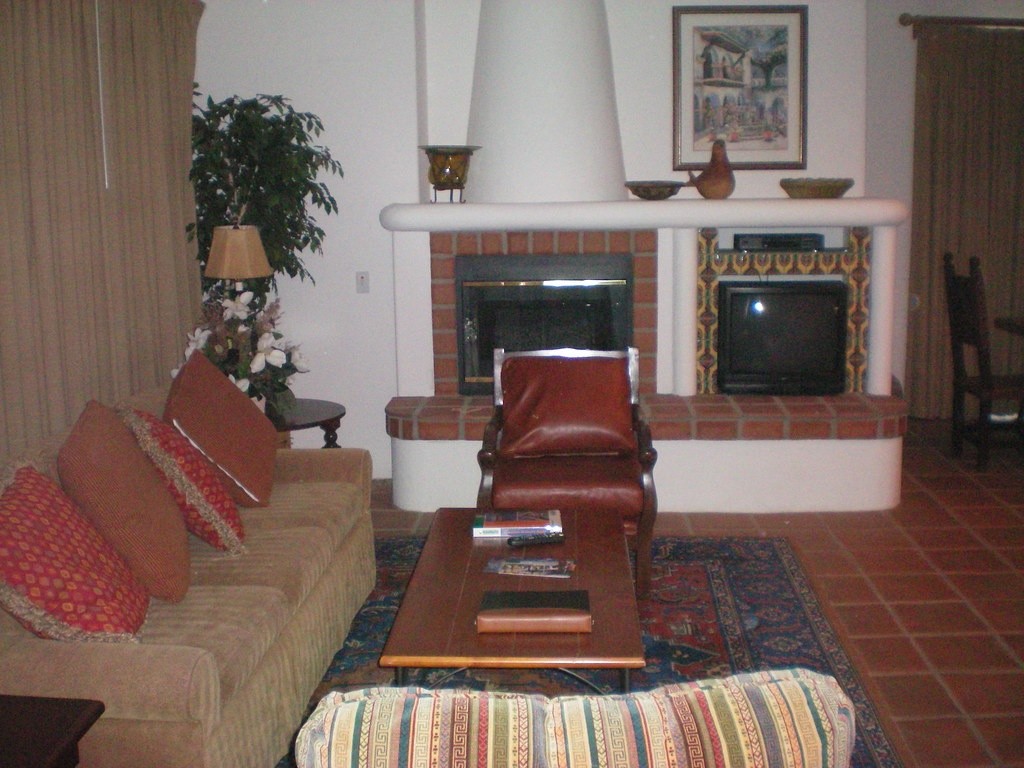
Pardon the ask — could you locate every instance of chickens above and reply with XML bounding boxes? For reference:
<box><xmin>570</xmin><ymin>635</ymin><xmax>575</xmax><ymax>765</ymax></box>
<box><xmin>688</xmin><ymin>139</ymin><xmax>736</xmax><ymax>199</ymax></box>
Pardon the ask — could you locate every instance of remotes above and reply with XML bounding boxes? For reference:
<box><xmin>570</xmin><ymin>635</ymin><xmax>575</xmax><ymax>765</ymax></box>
<box><xmin>507</xmin><ymin>532</ymin><xmax>565</xmax><ymax>547</ymax></box>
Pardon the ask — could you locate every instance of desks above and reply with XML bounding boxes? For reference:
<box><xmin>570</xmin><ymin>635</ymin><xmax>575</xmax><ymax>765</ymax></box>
<box><xmin>271</xmin><ymin>398</ymin><xmax>346</xmax><ymax>449</ymax></box>
<box><xmin>0</xmin><ymin>694</ymin><xmax>105</xmax><ymax>768</ymax></box>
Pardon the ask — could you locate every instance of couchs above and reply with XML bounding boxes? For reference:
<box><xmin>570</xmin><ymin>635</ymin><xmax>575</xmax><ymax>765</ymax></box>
<box><xmin>295</xmin><ymin>665</ymin><xmax>854</xmax><ymax>768</ymax></box>
<box><xmin>0</xmin><ymin>448</ymin><xmax>377</xmax><ymax>768</ymax></box>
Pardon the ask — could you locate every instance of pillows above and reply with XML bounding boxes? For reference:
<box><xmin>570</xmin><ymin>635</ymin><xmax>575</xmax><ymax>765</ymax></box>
<box><xmin>59</xmin><ymin>400</ymin><xmax>189</xmax><ymax>602</ymax></box>
<box><xmin>0</xmin><ymin>457</ymin><xmax>150</xmax><ymax>647</ymax></box>
<box><xmin>166</xmin><ymin>349</ymin><xmax>278</xmax><ymax>508</ymax></box>
<box><xmin>118</xmin><ymin>403</ymin><xmax>244</xmax><ymax>553</ymax></box>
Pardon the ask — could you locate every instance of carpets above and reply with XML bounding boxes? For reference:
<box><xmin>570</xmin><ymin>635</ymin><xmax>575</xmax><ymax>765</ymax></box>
<box><xmin>272</xmin><ymin>533</ymin><xmax>906</xmax><ymax>768</ymax></box>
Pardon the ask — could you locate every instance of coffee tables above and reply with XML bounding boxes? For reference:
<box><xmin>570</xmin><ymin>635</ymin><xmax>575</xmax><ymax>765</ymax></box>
<box><xmin>379</xmin><ymin>508</ymin><xmax>646</xmax><ymax>695</ymax></box>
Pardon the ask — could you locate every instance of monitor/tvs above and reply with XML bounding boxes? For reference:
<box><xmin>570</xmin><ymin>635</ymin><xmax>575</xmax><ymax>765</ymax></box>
<box><xmin>719</xmin><ymin>280</ymin><xmax>847</xmax><ymax>396</ymax></box>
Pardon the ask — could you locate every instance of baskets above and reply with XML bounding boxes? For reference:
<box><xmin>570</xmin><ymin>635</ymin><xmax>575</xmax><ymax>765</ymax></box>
<box><xmin>780</xmin><ymin>177</ymin><xmax>855</xmax><ymax>199</ymax></box>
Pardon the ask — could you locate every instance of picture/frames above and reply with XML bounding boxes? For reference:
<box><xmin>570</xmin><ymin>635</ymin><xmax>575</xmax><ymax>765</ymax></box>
<box><xmin>671</xmin><ymin>5</ymin><xmax>809</xmax><ymax>169</ymax></box>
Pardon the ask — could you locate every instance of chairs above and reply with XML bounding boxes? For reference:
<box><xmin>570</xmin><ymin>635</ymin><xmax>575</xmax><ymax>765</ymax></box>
<box><xmin>943</xmin><ymin>252</ymin><xmax>1024</xmax><ymax>472</ymax></box>
<box><xmin>476</xmin><ymin>347</ymin><xmax>658</xmax><ymax>593</ymax></box>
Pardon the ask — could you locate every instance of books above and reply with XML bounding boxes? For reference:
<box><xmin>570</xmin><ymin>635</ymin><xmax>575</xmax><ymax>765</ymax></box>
<box><xmin>473</xmin><ymin>510</ymin><xmax>563</xmax><ymax>538</ymax></box>
<box><xmin>478</xmin><ymin>590</ymin><xmax>592</xmax><ymax>632</ymax></box>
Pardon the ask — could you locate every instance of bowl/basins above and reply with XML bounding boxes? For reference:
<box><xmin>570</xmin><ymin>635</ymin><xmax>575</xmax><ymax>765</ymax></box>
<box><xmin>780</xmin><ymin>177</ymin><xmax>856</xmax><ymax>198</ymax></box>
<box><xmin>416</xmin><ymin>143</ymin><xmax>482</xmax><ymax>187</ymax></box>
<box><xmin>625</xmin><ymin>179</ymin><xmax>686</xmax><ymax>201</ymax></box>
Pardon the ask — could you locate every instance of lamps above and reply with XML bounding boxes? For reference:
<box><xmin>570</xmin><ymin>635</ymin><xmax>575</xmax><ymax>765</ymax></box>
<box><xmin>205</xmin><ymin>225</ymin><xmax>273</xmax><ymax>374</ymax></box>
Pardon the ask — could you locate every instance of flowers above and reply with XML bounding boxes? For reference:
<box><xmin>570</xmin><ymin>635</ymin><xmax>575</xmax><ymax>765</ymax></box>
<box><xmin>172</xmin><ymin>290</ymin><xmax>309</xmax><ymax>425</ymax></box>
